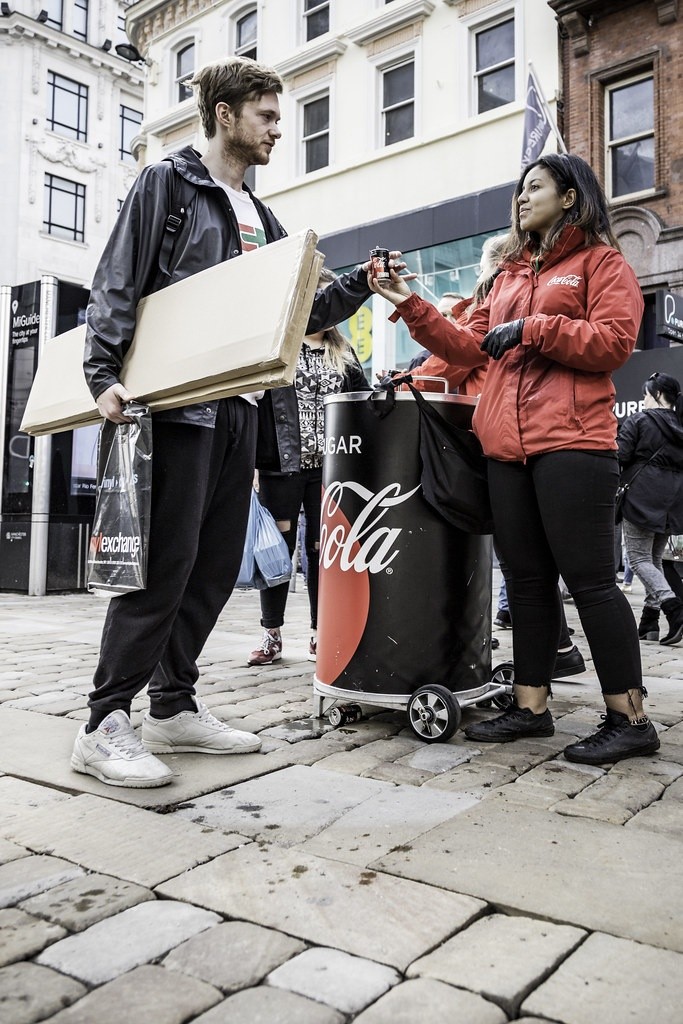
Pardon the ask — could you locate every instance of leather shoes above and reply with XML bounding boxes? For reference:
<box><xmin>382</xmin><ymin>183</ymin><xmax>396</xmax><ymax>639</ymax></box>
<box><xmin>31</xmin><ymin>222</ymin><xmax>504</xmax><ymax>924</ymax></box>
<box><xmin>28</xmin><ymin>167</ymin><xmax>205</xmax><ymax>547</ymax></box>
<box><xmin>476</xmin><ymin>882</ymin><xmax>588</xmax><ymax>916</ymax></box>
<box><xmin>551</xmin><ymin>645</ymin><xmax>586</xmax><ymax>679</ymax></box>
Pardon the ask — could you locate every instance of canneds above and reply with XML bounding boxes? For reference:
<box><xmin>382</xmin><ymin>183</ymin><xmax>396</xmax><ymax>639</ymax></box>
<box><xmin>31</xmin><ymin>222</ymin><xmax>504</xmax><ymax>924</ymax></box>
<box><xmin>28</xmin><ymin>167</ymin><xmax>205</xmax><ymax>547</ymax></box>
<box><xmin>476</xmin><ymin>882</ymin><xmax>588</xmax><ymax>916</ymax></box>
<box><xmin>369</xmin><ymin>246</ymin><xmax>391</xmax><ymax>285</ymax></box>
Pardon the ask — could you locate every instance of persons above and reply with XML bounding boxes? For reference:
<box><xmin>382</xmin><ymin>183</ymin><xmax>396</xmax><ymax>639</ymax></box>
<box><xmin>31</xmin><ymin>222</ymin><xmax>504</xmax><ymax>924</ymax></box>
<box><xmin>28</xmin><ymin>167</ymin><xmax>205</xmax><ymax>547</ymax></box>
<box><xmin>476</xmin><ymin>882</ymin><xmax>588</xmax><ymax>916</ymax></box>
<box><xmin>247</xmin><ymin>269</ymin><xmax>374</xmax><ymax>666</ymax></box>
<box><xmin>296</xmin><ymin>234</ymin><xmax>635</xmax><ymax>680</ymax></box>
<box><xmin>616</xmin><ymin>373</ymin><xmax>682</xmax><ymax>644</ymax></box>
<box><xmin>368</xmin><ymin>153</ymin><xmax>660</xmax><ymax>765</ymax></box>
<box><xmin>71</xmin><ymin>57</ymin><xmax>416</xmax><ymax>788</ymax></box>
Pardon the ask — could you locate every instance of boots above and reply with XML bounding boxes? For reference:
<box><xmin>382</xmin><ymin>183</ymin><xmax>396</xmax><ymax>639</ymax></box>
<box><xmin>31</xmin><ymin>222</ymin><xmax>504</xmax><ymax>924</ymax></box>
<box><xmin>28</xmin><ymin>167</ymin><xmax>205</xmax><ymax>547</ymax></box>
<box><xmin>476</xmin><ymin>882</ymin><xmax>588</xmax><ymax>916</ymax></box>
<box><xmin>660</xmin><ymin>596</ymin><xmax>683</xmax><ymax>645</ymax></box>
<box><xmin>637</xmin><ymin>605</ymin><xmax>661</xmax><ymax>641</ymax></box>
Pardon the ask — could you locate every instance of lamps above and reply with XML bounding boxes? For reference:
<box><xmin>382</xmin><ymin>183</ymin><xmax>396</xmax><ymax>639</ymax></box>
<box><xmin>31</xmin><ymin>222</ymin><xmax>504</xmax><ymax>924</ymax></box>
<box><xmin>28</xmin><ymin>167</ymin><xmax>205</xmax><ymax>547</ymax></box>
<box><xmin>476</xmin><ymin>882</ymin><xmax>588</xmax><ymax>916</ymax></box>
<box><xmin>115</xmin><ymin>44</ymin><xmax>151</xmax><ymax>66</ymax></box>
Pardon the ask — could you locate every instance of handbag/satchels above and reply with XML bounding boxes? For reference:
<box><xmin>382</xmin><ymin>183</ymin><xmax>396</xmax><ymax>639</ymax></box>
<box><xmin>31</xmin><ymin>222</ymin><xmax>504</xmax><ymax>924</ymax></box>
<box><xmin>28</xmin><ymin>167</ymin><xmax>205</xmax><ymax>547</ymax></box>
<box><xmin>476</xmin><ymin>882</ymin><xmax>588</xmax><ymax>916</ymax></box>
<box><xmin>230</xmin><ymin>485</ymin><xmax>292</xmax><ymax>591</ymax></box>
<box><xmin>614</xmin><ymin>486</ymin><xmax>628</xmax><ymax>526</ymax></box>
<box><xmin>415</xmin><ymin>400</ymin><xmax>492</xmax><ymax>535</ymax></box>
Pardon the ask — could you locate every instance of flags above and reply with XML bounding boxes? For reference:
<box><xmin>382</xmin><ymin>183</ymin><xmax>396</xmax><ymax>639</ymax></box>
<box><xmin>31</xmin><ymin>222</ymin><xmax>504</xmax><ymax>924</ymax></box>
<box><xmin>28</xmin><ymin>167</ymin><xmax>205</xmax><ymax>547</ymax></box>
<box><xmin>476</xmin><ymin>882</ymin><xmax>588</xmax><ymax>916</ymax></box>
<box><xmin>520</xmin><ymin>69</ymin><xmax>553</xmax><ymax>176</ymax></box>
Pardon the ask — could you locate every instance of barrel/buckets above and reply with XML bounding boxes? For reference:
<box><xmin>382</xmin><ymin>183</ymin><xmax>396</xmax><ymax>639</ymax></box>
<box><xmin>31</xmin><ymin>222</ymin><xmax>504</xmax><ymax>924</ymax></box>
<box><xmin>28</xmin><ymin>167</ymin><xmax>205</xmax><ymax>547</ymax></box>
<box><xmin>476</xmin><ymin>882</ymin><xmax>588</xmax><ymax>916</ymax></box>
<box><xmin>313</xmin><ymin>377</ymin><xmax>495</xmax><ymax>705</ymax></box>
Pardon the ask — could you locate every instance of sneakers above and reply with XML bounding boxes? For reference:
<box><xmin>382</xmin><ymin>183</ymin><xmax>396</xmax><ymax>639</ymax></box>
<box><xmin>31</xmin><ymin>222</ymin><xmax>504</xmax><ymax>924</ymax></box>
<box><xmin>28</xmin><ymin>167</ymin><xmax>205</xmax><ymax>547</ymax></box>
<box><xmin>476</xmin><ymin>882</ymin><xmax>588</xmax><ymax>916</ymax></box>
<box><xmin>246</xmin><ymin>633</ymin><xmax>282</xmax><ymax>665</ymax></box>
<box><xmin>465</xmin><ymin>695</ymin><xmax>554</xmax><ymax>743</ymax></box>
<box><xmin>307</xmin><ymin>636</ymin><xmax>317</xmax><ymax>661</ymax></box>
<box><xmin>71</xmin><ymin>709</ymin><xmax>173</xmax><ymax>789</ymax></box>
<box><xmin>564</xmin><ymin>706</ymin><xmax>660</xmax><ymax>764</ymax></box>
<box><xmin>141</xmin><ymin>696</ymin><xmax>262</xmax><ymax>754</ymax></box>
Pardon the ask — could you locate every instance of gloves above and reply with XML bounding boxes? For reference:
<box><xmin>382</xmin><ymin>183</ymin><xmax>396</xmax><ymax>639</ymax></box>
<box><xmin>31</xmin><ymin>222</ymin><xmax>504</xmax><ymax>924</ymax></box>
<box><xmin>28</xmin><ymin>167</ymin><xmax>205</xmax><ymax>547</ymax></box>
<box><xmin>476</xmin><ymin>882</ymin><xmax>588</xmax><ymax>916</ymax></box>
<box><xmin>480</xmin><ymin>317</ymin><xmax>524</xmax><ymax>360</ymax></box>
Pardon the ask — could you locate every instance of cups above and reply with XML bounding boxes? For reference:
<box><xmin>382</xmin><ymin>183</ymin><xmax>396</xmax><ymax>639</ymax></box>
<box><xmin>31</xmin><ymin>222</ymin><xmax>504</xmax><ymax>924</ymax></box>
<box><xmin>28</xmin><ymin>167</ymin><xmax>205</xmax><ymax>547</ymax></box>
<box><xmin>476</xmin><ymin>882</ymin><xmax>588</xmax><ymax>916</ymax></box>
<box><xmin>369</xmin><ymin>248</ymin><xmax>391</xmax><ymax>286</ymax></box>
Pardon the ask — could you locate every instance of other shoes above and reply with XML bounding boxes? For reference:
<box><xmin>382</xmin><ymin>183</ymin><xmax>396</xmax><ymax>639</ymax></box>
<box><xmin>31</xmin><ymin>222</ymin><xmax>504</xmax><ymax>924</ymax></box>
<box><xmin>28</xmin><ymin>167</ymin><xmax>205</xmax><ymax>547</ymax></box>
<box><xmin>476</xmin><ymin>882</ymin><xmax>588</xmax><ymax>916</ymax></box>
<box><xmin>562</xmin><ymin>589</ymin><xmax>573</xmax><ymax>600</ymax></box>
<box><xmin>620</xmin><ymin>584</ymin><xmax>632</xmax><ymax>592</ymax></box>
<box><xmin>493</xmin><ymin>609</ymin><xmax>513</xmax><ymax>628</ymax></box>
<box><xmin>618</xmin><ymin>566</ymin><xmax>626</xmax><ymax>572</ymax></box>
<box><xmin>615</xmin><ymin>575</ymin><xmax>624</xmax><ymax>583</ymax></box>
<box><xmin>568</xmin><ymin>627</ymin><xmax>575</xmax><ymax>634</ymax></box>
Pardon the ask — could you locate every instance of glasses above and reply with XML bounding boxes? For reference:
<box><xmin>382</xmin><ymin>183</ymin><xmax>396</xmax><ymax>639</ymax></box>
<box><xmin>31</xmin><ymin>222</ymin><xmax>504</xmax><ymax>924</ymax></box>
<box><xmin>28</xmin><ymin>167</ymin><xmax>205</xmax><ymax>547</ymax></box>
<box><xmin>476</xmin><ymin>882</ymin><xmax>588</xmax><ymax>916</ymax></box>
<box><xmin>648</xmin><ymin>372</ymin><xmax>661</xmax><ymax>392</ymax></box>
